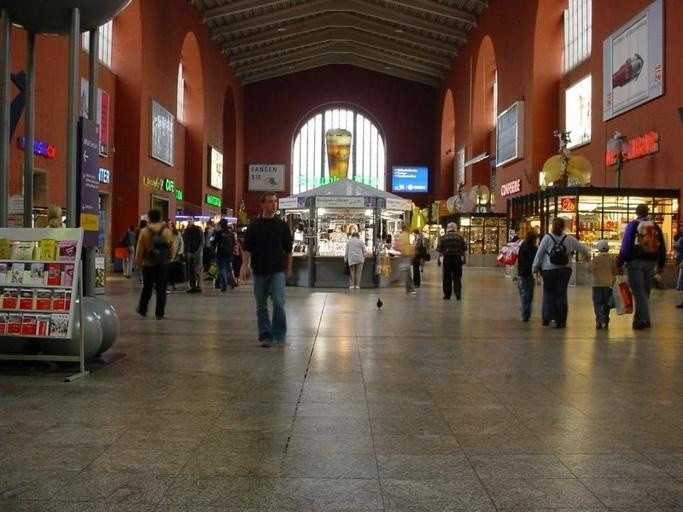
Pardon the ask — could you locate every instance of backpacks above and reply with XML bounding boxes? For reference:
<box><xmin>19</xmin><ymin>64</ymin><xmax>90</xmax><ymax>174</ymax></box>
<box><xmin>144</xmin><ymin>224</ymin><xmax>171</xmax><ymax>263</ymax></box>
<box><xmin>544</xmin><ymin>233</ymin><xmax>569</xmax><ymax>266</ymax></box>
<box><xmin>633</xmin><ymin>219</ymin><xmax>660</xmax><ymax>253</ymax></box>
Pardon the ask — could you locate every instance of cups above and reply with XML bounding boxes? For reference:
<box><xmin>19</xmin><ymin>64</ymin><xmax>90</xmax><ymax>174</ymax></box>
<box><xmin>325</xmin><ymin>128</ymin><xmax>351</xmax><ymax>180</ymax></box>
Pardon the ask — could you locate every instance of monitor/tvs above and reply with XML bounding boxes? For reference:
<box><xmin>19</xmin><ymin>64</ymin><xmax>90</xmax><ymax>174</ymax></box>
<box><xmin>391</xmin><ymin>164</ymin><xmax>429</xmax><ymax>194</ymax></box>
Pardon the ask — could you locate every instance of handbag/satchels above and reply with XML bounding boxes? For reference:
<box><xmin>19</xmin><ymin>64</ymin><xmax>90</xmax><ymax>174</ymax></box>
<box><xmin>342</xmin><ymin>263</ymin><xmax>351</xmax><ymax>275</ymax></box>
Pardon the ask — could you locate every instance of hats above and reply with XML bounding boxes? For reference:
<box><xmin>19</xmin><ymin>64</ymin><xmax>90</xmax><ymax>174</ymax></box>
<box><xmin>597</xmin><ymin>240</ymin><xmax>609</xmax><ymax>252</ymax></box>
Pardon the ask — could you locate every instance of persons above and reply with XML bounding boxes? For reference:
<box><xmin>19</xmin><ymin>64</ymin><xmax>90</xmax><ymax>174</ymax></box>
<box><xmin>512</xmin><ymin>230</ymin><xmax>541</xmax><ymax>321</ymax></box>
<box><xmin>583</xmin><ymin>240</ymin><xmax>616</xmax><ymax>329</ymax></box>
<box><xmin>673</xmin><ymin>232</ymin><xmax>683</xmax><ymax>308</ymax></box>
<box><xmin>123</xmin><ymin>208</ymin><xmax>243</xmax><ymax>320</ymax></box>
<box><xmin>616</xmin><ymin>204</ymin><xmax>666</xmax><ymax>329</ymax></box>
<box><xmin>436</xmin><ymin>222</ymin><xmax>467</xmax><ymax>300</ymax></box>
<box><xmin>344</xmin><ymin>232</ymin><xmax>367</xmax><ymax>289</ymax></box>
<box><xmin>532</xmin><ymin>218</ymin><xmax>588</xmax><ymax>328</ymax></box>
<box><xmin>242</xmin><ymin>192</ymin><xmax>293</xmax><ymax>347</ymax></box>
<box><xmin>386</xmin><ymin>229</ymin><xmax>427</xmax><ymax>294</ymax></box>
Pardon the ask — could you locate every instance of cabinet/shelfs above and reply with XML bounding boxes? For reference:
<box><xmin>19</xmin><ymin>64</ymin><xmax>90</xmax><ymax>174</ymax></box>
<box><xmin>0</xmin><ymin>227</ymin><xmax>91</xmax><ymax>383</ymax></box>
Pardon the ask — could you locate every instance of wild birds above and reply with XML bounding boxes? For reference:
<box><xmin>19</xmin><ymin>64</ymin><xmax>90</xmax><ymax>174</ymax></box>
<box><xmin>377</xmin><ymin>297</ymin><xmax>382</xmax><ymax>308</ymax></box>
